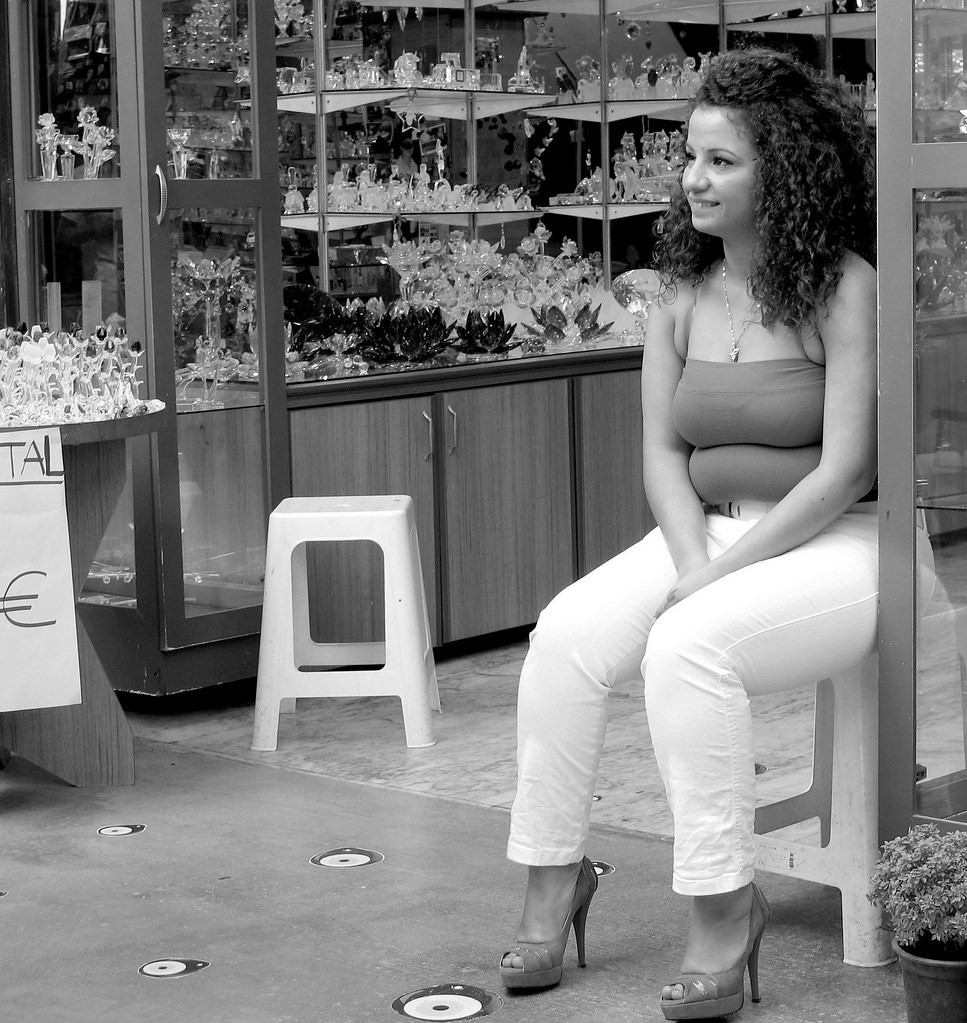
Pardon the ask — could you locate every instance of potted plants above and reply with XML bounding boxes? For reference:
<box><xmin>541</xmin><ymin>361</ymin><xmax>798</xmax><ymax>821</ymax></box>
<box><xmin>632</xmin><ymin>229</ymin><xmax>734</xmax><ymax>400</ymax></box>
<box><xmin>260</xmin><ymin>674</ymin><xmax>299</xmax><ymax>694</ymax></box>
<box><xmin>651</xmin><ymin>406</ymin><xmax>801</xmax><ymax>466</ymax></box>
<box><xmin>866</xmin><ymin>821</ymin><xmax>967</xmax><ymax>1023</ymax></box>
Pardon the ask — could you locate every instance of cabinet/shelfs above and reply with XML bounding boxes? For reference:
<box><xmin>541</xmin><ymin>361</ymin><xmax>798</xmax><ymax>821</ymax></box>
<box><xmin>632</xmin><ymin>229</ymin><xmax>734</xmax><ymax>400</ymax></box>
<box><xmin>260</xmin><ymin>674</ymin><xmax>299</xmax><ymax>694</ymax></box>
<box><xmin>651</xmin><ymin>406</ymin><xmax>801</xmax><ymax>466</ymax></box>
<box><xmin>36</xmin><ymin>1</ymin><xmax>967</xmax><ymax>694</ymax></box>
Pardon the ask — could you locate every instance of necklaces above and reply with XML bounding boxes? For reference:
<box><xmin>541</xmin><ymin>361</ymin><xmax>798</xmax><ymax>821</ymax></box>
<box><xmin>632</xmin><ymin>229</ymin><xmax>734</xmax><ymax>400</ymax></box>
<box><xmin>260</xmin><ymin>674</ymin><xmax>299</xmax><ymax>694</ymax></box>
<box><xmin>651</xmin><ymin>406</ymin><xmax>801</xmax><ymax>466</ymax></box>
<box><xmin>723</xmin><ymin>259</ymin><xmax>764</xmax><ymax>363</ymax></box>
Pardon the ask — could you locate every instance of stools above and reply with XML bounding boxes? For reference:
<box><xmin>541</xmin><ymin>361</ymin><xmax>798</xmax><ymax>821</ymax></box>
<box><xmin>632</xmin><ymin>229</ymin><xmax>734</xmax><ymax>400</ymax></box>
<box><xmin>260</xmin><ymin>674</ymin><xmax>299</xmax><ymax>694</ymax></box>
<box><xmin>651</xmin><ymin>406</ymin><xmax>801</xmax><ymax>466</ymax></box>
<box><xmin>250</xmin><ymin>494</ymin><xmax>441</xmax><ymax>752</ymax></box>
<box><xmin>751</xmin><ymin>600</ymin><xmax>966</xmax><ymax>969</ymax></box>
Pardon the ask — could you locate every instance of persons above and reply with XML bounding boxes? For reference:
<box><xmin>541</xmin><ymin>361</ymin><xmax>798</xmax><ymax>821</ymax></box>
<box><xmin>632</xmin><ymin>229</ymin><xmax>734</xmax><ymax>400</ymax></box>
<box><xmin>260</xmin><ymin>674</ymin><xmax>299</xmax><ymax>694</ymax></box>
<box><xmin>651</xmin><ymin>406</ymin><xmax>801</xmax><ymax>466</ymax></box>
<box><xmin>499</xmin><ymin>47</ymin><xmax>933</xmax><ymax>1021</ymax></box>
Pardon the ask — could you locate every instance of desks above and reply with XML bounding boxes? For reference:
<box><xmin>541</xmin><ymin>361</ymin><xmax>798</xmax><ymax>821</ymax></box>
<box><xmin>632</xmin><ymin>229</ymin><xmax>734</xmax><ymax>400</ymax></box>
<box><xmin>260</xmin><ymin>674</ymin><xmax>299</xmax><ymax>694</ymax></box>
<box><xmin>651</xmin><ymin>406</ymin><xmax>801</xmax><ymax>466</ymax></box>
<box><xmin>0</xmin><ymin>398</ymin><xmax>166</xmax><ymax>787</ymax></box>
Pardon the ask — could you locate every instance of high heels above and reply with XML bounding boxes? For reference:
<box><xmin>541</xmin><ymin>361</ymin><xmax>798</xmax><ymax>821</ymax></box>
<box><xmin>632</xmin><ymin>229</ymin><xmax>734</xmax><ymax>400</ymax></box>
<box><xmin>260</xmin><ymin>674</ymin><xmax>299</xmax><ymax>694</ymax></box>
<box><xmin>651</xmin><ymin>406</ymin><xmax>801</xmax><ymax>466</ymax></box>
<box><xmin>659</xmin><ymin>882</ymin><xmax>771</xmax><ymax>1020</ymax></box>
<box><xmin>499</xmin><ymin>855</ymin><xmax>599</xmax><ymax>988</ymax></box>
